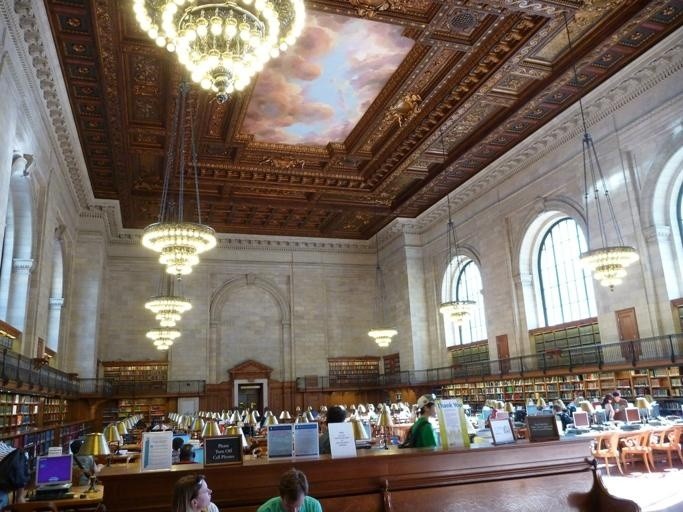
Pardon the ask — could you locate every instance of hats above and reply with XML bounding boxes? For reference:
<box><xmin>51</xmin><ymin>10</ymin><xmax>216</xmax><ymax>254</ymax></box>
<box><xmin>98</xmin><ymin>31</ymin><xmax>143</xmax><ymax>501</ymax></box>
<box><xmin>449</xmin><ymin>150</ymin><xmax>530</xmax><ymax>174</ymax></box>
<box><xmin>418</xmin><ymin>394</ymin><xmax>436</xmax><ymax>409</ymax></box>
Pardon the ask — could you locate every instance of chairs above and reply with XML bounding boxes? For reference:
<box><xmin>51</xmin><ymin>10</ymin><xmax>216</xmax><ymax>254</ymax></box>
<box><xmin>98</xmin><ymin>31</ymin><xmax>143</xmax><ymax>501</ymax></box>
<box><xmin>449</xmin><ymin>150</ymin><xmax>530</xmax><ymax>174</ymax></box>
<box><xmin>650</xmin><ymin>425</ymin><xmax>683</xmax><ymax>466</ymax></box>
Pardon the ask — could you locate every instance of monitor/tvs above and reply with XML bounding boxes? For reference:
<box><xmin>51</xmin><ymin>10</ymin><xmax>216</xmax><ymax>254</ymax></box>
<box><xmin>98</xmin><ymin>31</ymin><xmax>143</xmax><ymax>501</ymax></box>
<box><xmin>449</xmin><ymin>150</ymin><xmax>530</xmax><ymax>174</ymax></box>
<box><xmin>36</xmin><ymin>454</ymin><xmax>73</xmax><ymax>486</ymax></box>
<box><xmin>172</xmin><ymin>434</ymin><xmax>203</xmax><ymax>465</ymax></box>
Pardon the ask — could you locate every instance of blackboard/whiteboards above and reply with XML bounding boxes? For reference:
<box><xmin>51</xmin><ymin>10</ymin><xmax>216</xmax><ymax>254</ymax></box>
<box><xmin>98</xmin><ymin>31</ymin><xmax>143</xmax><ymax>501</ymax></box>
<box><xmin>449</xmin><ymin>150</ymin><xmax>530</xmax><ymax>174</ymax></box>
<box><xmin>527</xmin><ymin>415</ymin><xmax>560</xmax><ymax>443</ymax></box>
<box><xmin>203</xmin><ymin>435</ymin><xmax>244</xmax><ymax>467</ymax></box>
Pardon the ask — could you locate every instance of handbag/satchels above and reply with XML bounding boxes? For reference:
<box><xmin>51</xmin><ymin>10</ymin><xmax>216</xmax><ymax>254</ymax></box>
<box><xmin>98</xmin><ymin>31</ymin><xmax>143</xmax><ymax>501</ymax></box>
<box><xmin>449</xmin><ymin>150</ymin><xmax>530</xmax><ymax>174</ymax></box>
<box><xmin>0</xmin><ymin>449</ymin><xmax>30</xmax><ymax>492</ymax></box>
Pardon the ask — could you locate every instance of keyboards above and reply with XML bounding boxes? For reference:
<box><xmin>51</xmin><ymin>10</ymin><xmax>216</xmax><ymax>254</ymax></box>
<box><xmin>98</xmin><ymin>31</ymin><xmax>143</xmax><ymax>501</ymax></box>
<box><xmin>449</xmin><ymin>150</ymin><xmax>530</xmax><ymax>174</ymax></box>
<box><xmin>29</xmin><ymin>493</ymin><xmax>74</xmax><ymax>502</ymax></box>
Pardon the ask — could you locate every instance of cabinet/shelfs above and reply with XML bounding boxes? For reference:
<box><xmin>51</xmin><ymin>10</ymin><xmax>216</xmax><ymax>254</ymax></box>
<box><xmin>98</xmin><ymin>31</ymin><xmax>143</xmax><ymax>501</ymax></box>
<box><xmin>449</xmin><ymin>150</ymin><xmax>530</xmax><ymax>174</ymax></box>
<box><xmin>0</xmin><ymin>387</ymin><xmax>85</xmax><ymax>512</ymax></box>
<box><xmin>434</xmin><ymin>298</ymin><xmax>683</xmax><ymax>416</ymax></box>
<box><xmin>101</xmin><ymin>360</ymin><xmax>169</xmax><ymax>423</ymax></box>
<box><xmin>328</xmin><ymin>353</ymin><xmax>400</xmax><ymax>386</ymax></box>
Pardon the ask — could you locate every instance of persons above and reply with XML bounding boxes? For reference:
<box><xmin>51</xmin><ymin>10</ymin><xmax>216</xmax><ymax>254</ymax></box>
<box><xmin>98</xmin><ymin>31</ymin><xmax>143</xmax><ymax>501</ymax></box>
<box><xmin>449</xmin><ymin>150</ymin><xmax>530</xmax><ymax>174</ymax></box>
<box><xmin>152</xmin><ymin>421</ymin><xmax>199</xmax><ymax>464</ymax></box>
<box><xmin>319</xmin><ymin>407</ymin><xmax>346</xmax><ymax>453</ymax></box>
<box><xmin>256</xmin><ymin>470</ymin><xmax>322</xmax><ymax>512</ymax></box>
<box><xmin>548</xmin><ymin>391</ymin><xmax>629</xmax><ymax>431</ymax></box>
<box><xmin>170</xmin><ymin>475</ymin><xmax>219</xmax><ymax>512</ymax></box>
<box><xmin>0</xmin><ymin>441</ymin><xmax>28</xmax><ymax>512</ymax></box>
<box><xmin>398</xmin><ymin>393</ymin><xmax>442</xmax><ymax>449</ymax></box>
<box><xmin>69</xmin><ymin>440</ymin><xmax>103</xmax><ymax>485</ymax></box>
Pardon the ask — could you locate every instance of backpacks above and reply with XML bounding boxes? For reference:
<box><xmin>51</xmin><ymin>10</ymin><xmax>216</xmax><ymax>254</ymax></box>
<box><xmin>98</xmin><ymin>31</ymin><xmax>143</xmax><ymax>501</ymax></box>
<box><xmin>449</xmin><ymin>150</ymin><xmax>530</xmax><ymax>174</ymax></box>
<box><xmin>398</xmin><ymin>422</ymin><xmax>429</xmax><ymax>448</ymax></box>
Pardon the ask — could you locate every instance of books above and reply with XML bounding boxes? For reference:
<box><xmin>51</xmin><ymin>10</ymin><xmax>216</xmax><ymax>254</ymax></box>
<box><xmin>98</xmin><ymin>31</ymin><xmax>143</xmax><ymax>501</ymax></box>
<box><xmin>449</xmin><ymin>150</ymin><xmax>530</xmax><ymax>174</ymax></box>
<box><xmin>0</xmin><ymin>394</ymin><xmax>95</xmax><ymax>469</ymax></box>
<box><xmin>103</xmin><ymin>399</ymin><xmax>168</xmax><ymax>433</ymax></box>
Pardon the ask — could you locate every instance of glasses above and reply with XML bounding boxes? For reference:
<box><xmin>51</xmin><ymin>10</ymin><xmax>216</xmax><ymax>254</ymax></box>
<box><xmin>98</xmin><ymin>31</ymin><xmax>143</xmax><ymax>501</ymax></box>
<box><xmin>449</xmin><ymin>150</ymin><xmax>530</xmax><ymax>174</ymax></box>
<box><xmin>195</xmin><ymin>477</ymin><xmax>202</xmax><ymax>488</ymax></box>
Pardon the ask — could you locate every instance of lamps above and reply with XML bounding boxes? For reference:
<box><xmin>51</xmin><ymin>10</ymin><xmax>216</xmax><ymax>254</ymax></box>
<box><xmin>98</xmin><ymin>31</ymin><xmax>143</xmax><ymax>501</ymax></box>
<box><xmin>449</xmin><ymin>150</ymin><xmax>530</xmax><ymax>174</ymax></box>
<box><xmin>131</xmin><ymin>0</ymin><xmax>305</xmax><ymax>106</ymax></box>
<box><xmin>560</xmin><ymin>11</ymin><xmax>639</xmax><ymax>293</ymax></box>
<box><xmin>140</xmin><ymin>64</ymin><xmax>217</xmax><ymax>281</ymax></box>
<box><xmin>144</xmin><ymin>264</ymin><xmax>193</xmax><ymax>329</ymax></box>
<box><xmin>367</xmin><ymin>194</ymin><xmax>398</xmax><ymax>349</ymax></box>
<box><xmin>145</xmin><ymin>330</ymin><xmax>182</xmax><ymax>353</ymax></box>
<box><xmin>438</xmin><ymin>128</ymin><xmax>476</xmax><ymax>325</ymax></box>
